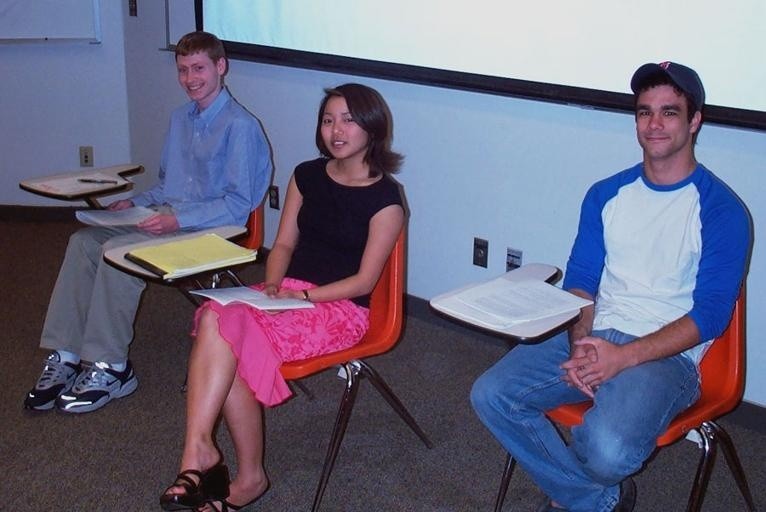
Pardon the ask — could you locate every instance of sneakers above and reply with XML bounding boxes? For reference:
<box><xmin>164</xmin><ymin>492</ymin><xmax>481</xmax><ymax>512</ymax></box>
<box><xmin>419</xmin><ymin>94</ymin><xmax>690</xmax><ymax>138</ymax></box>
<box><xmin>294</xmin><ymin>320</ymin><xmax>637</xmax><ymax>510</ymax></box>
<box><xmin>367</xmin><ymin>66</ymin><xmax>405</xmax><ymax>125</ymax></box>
<box><xmin>53</xmin><ymin>358</ymin><xmax>138</xmax><ymax>413</ymax></box>
<box><xmin>22</xmin><ymin>350</ymin><xmax>83</xmax><ymax>412</ymax></box>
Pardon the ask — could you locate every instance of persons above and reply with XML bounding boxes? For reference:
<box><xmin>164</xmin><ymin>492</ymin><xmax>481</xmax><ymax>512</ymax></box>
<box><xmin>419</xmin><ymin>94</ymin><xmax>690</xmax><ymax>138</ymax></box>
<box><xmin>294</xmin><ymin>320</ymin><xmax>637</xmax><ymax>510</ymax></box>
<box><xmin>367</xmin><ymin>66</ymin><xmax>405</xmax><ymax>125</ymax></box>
<box><xmin>470</xmin><ymin>62</ymin><xmax>751</xmax><ymax>512</ymax></box>
<box><xmin>25</xmin><ymin>31</ymin><xmax>274</xmax><ymax>415</ymax></box>
<box><xmin>160</xmin><ymin>84</ymin><xmax>406</xmax><ymax>512</ymax></box>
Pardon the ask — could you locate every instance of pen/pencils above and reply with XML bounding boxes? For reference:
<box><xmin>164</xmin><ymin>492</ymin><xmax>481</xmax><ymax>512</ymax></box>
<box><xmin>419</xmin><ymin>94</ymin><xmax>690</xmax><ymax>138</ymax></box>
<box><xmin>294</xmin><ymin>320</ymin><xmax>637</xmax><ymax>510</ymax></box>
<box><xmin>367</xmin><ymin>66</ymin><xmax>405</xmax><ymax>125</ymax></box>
<box><xmin>77</xmin><ymin>179</ymin><xmax>118</xmax><ymax>183</ymax></box>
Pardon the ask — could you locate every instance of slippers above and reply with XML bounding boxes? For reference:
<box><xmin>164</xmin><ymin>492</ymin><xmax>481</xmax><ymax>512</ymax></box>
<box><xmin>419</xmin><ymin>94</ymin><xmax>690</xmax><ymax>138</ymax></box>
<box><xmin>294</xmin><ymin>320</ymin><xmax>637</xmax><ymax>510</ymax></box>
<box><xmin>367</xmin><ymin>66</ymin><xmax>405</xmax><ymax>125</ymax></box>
<box><xmin>158</xmin><ymin>462</ymin><xmax>231</xmax><ymax>509</ymax></box>
<box><xmin>194</xmin><ymin>470</ymin><xmax>271</xmax><ymax>512</ymax></box>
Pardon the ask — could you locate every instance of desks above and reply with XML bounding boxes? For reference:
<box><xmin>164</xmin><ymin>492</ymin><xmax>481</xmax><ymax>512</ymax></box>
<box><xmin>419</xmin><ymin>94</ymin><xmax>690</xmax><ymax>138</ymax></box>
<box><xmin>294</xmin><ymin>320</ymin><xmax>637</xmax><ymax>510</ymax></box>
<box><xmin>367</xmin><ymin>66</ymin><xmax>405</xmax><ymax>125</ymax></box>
<box><xmin>427</xmin><ymin>260</ymin><xmax>584</xmax><ymax>341</ymax></box>
<box><xmin>19</xmin><ymin>163</ymin><xmax>145</xmax><ymax>203</ymax></box>
<box><xmin>100</xmin><ymin>224</ymin><xmax>257</xmax><ymax>284</ymax></box>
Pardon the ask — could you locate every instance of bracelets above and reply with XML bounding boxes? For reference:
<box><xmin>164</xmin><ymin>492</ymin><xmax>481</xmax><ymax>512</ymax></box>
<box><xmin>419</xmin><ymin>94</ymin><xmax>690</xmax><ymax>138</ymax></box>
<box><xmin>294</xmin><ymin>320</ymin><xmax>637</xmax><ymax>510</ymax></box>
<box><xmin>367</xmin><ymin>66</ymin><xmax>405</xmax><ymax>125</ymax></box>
<box><xmin>302</xmin><ymin>290</ymin><xmax>310</xmax><ymax>301</ymax></box>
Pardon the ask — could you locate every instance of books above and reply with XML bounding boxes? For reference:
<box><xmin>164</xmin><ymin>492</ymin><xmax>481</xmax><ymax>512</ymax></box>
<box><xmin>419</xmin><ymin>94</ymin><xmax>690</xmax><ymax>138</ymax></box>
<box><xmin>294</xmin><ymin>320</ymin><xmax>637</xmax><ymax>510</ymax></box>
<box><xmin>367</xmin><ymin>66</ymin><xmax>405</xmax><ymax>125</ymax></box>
<box><xmin>123</xmin><ymin>232</ymin><xmax>257</xmax><ymax>280</ymax></box>
<box><xmin>189</xmin><ymin>286</ymin><xmax>316</xmax><ymax>311</ymax></box>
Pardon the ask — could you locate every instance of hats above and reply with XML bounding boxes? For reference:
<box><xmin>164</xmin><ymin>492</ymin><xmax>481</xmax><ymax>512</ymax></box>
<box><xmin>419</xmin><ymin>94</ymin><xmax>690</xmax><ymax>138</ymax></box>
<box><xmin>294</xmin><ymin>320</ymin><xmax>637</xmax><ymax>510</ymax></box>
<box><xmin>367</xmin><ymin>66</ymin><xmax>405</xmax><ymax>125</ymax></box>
<box><xmin>629</xmin><ymin>60</ymin><xmax>704</xmax><ymax>113</ymax></box>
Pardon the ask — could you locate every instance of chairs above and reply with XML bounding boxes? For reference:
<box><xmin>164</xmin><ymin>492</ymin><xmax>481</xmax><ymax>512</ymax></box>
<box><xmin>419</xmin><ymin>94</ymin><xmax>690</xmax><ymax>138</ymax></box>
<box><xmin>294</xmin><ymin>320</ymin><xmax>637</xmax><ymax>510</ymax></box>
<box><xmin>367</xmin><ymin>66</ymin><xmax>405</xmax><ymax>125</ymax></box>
<box><xmin>489</xmin><ymin>277</ymin><xmax>758</xmax><ymax>511</ymax></box>
<box><xmin>221</xmin><ymin>203</ymin><xmax>434</xmax><ymax>511</ymax></box>
<box><xmin>176</xmin><ymin>193</ymin><xmax>317</xmax><ymax>394</ymax></box>
